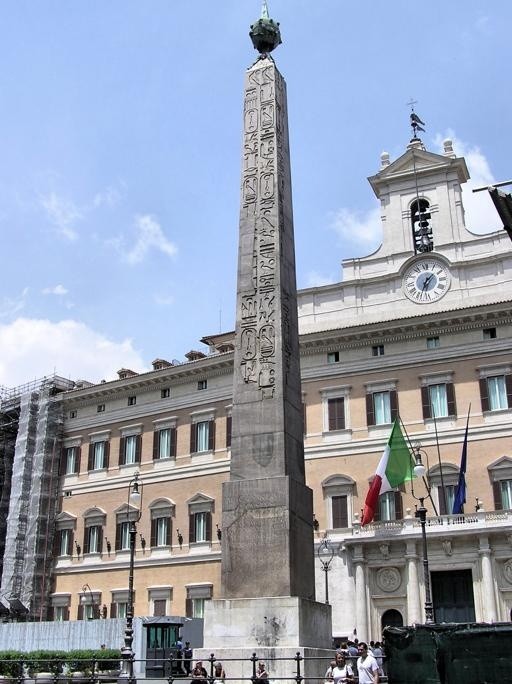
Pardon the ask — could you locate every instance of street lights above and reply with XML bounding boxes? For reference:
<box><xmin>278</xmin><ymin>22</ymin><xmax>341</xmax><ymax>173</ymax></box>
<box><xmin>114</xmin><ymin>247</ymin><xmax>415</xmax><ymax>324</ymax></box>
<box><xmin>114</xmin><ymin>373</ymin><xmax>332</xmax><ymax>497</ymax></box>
<box><xmin>318</xmin><ymin>538</ymin><xmax>336</xmax><ymax>605</ymax></box>
<box><xmin>115</xmin><ymin>468</ymin><xmax>146</xmax><ymax>683</ymax></box>
<box><xmin>408</xmin><ymin>439</ymin><xmax>435</xmax><ymax>625</ymax></box>
<box><xmin>82</xmin><ymin>582</ymin><xmax>97</xmax><ymax>621</ymax></box>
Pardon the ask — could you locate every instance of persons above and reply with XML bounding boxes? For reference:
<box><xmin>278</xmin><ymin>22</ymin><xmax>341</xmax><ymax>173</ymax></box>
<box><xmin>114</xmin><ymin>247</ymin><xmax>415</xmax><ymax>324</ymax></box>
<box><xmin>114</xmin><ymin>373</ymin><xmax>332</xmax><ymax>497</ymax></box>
<box><xmin>180</xmin><ymin>641</ymin><xmax>194</xmax><ymax>678</ymax></box>
<box><xmin>100</xmin><ymin>603</ymin><xmax>108</xmax><ymax>618</ymax></box>
<box><xmin>255</xmin><ymin>660</ymin><xmax>269</xmax><ymax>679</ymax></box>
<box><xmin>209</xmin><ymin>662</ymin><xmax>226</xmax><ymax>684</ymax></box>
<box><xmin>190</xmin><ymin>661</ymin><xmax>208</xmax><ymax>683</ymax></box>
<box><xmin>323</xmin><ymin>637</ymin><xmax>386</xmax><ymax>684</ymax></box>
<box><xmin>174</xmin><ymin>635</ymin><xmax>185</xmax><ymax>674</ymax></box>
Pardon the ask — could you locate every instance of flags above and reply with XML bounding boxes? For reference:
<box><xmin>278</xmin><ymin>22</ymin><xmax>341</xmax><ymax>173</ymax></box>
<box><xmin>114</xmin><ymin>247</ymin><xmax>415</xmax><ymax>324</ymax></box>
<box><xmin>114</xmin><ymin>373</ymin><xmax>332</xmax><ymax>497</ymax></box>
<box><xmin>451</xmin><ymin>402</ymin><xmax>476</xmax><ymax>515</ymax></box>
<box><xmin>357</xmin><ymin>411</ymin><xmax>420</xmax><ymax>527</ymax></box>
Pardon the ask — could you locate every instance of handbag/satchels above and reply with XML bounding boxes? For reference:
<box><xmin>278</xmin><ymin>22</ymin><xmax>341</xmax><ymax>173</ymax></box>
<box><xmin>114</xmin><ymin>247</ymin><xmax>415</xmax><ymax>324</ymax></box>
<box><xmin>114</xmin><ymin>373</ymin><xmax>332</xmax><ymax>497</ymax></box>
<box><xmin>346</xmin><ymin>672</ymin><xmax>356</xmax><ymax>684</ymax></box>
